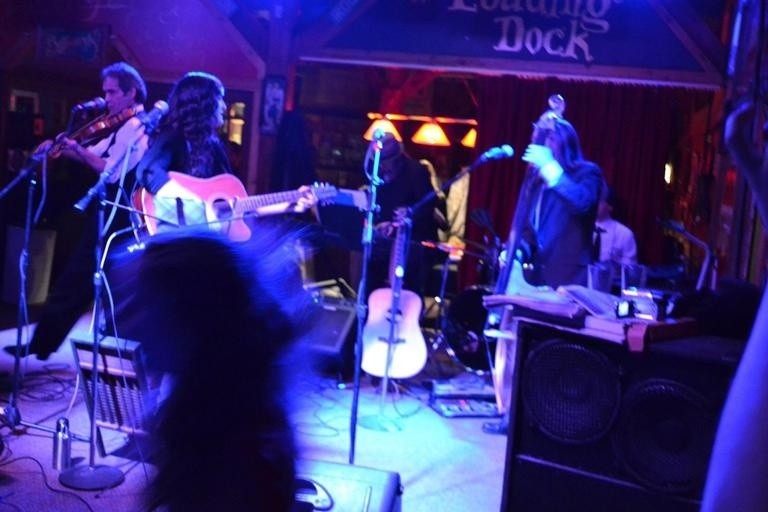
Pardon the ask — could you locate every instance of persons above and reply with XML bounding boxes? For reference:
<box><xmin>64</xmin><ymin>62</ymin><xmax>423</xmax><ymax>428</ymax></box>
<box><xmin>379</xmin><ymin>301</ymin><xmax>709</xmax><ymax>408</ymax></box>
<box><xmin>133</xmin><ymin>229</ymin><xmax>301</xmax><ymax>511</ymax></box>
<box><xmin>588</xmin><ymin>184</ymin><xmax>639</xmax><ymax>296</ymax></box>
<box><xmin>259</xmin><ymin>73</ymin><xmax>287</xmax><ymax>135</ymax></box>
<box><xmin>2</xmin><ymin>60</ymin><xmax>152</xmax><ymax>361</ymax></box>
<box><xmin>363</xmin><ymin>130</ymin><xmax>439</xmax><ymax>295</ymax></box>
<box><xmin>135</xmin><ymin>70</ymin><xmax>320</xmax><ymax>245</ymax></box>
<box><xmin>481</xmin><ymin>118</ymin><xmax>605</xmax><ymax>435</ymax></box>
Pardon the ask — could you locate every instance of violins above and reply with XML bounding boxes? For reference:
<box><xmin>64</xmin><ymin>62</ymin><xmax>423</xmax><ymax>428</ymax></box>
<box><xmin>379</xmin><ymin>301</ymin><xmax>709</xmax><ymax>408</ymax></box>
<box><xmin>51</xmin><ymin>108</ymin><xmax>135</xmax><ymax>155</ymax></box>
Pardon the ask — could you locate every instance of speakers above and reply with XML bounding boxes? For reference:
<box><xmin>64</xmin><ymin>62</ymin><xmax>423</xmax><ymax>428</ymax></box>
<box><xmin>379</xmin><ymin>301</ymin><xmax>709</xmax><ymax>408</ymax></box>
<box><xmin>300</xmin><ymin>302</ymin><xmax>358</xmax><ymax>371</ymax></box>
<box><xmin>500</xmin><ymin>310</ymin><xmax>741</xmax><ymax>512</ymax></box>
<box><xmin>285</xmin><ymin>458</ymin><xmax>405</xmax><ymax>512</ymax></box>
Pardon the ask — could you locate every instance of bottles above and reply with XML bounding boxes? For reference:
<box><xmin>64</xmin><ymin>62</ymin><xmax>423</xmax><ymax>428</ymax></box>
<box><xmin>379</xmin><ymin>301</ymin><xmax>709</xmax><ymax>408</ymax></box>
<box><xmin>53</xmin><ymin>418</ymin><xmax>70</xmax><ymax>472</ymax></box>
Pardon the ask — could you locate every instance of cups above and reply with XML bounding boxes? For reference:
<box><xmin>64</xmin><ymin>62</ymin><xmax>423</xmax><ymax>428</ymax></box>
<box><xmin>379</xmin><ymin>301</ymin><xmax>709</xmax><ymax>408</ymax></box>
<box><xmin>587</xmin><ymin>262</ymin><xmax>646</xmax><ymax>293</ymax></box>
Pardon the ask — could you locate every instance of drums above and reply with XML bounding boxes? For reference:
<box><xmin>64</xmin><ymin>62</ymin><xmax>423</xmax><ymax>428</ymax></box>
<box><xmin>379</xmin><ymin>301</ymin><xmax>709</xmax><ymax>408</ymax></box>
<box><xmin>442</xmin><ymin>285</ymin><xmax>502</xmax><ymax>375</ymax></box>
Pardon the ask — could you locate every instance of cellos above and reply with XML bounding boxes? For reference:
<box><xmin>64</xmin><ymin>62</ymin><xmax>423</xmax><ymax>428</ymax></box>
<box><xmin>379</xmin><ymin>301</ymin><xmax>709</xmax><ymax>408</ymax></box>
<box><xmin>483</xmin><ymin>94</ymin><xmax>565</xmax><ymax>414</ymax></box>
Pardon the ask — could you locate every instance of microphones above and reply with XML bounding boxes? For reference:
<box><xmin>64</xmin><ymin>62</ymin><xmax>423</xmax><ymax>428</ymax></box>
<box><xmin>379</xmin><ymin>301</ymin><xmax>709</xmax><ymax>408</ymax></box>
<box><xmin>484</xmin><ymin>142</ymin><xmax>516</xmax><ymax>161</ymax></box>
<box><xmin>77</xmin><ymin>96</ymin><xmax>106</xmax><ymax>115</ymax></box>
<box><xmin>136</xmin><ymin>98</ymin><xmax>170</xmax><ymax>131</ymax></box>
<box><xmin>369</xmin><ymin>127</ymin><xmax>388</xmax><ymax>142</ymax></box>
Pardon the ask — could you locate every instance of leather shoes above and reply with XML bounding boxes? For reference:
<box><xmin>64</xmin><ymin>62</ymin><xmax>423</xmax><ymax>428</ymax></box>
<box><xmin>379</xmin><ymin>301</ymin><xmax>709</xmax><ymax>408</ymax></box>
<box><xmin>3</xmin><ymin>343</ymin><xmax>53</xmax><ymax>361</ymax></box>
<box><xmin>483</xmin><ymin>418</ymin><xmax>510</xmax><ymax>435</ymax></box>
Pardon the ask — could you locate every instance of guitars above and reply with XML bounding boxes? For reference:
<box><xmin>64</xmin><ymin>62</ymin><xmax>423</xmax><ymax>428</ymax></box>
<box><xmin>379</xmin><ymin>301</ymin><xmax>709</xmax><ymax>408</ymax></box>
<box><xmin>133</xmin><ymin>171</ymin><xmax>338</xmax><ymax>243</ymax></box>
<box><xmin>355</xmin><ymin>208</ymin><xmax>429</xmax><ymax>379</ymax></box>
